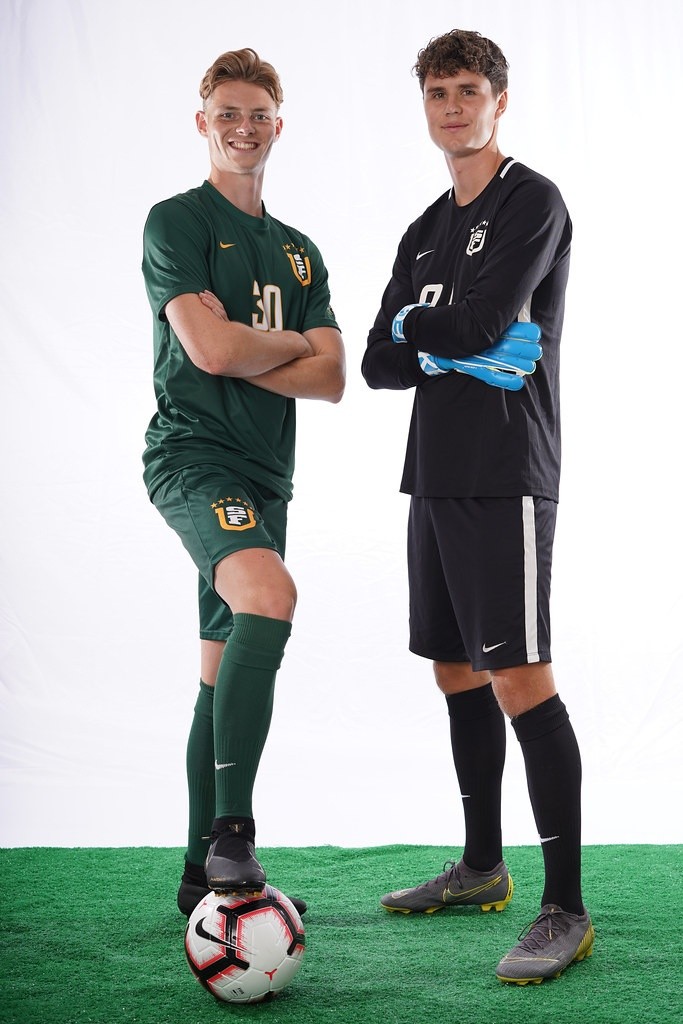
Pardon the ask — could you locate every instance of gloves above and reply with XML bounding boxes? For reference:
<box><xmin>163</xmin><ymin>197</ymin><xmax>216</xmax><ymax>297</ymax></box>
<box><xmin>417</xmin><ymin>321</ymin><xmax>542</xmax><ymax>391</ymax></box>
<box><xmin>392</xmin><ymin>302</ymin><xmax>422</xmax><ymax>341</ymax></box>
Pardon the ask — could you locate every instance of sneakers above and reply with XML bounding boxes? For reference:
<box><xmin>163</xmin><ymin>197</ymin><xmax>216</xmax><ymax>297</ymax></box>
<box><xmin>175</xmin><ymin>861</ymin><xmax>310</xmax><ymax>917</ymax></box>
<box><xmin>379</xmin><ymin>859</ymin><xmax>514</xmax><ymax>914</ymax></box>
<box><xmin>496</xmin><ymin>903</ymin><xmax>597</xmax><ymax>985</ymax></box>
<box><xmin>203</xmin><ymin>816</ymin><xmax>266</xmax><ymax>893</ymax></box>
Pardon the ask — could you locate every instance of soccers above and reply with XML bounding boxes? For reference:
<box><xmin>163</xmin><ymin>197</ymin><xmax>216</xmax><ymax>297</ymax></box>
<box><xmin>185</xmin><ymin>883</ymin><xmax>305</xmax><ymax>1008</ymax></box>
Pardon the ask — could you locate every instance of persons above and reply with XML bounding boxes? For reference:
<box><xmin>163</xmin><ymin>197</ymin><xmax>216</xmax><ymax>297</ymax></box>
<box><xmin>142</xmin><ymin>47</ymin><xmax>346</xmax><ymax>920</ymax></box>
<box><xmin>360</xmin><ymin>29</ymin><xmax>594</xmax><ymax>984</ymax></box>
